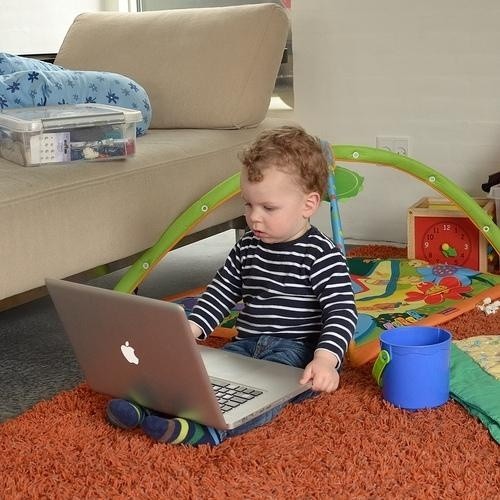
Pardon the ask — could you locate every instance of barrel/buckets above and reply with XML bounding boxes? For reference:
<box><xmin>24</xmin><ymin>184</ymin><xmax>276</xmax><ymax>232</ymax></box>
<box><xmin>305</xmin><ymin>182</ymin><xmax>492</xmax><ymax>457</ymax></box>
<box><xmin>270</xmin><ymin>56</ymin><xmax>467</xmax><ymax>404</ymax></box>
<box><xmin>378</xmin><ymin>325</ymin><xmax>452</xmax><ymax>412</ymax></box>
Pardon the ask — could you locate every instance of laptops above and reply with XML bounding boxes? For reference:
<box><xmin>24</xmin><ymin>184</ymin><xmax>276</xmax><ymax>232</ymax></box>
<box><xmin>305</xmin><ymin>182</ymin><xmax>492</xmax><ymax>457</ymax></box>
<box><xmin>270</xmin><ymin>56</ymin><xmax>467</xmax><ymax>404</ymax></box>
<box><xmin>44</xmin><ymin>276</ymin><xmax>314</xmax><ymax>433</ymax></box>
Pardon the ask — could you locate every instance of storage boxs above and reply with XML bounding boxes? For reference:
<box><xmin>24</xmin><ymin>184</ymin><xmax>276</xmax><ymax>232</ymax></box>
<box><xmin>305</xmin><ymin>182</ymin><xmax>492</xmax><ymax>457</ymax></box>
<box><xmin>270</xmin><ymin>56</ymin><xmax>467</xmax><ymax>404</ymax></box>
<box><xmin>0</xmin><ymin>102</ymin><xmax>143</xmax><ymax>168</ymax></box>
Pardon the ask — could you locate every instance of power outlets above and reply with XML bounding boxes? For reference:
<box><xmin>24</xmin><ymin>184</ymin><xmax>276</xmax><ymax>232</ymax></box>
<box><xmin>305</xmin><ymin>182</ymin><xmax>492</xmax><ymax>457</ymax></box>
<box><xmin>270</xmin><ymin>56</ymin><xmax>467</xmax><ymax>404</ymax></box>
<box><xmin>378</xmin><ymin>138</ymin><xmax>410</xmax><ymax>167</ymax></box>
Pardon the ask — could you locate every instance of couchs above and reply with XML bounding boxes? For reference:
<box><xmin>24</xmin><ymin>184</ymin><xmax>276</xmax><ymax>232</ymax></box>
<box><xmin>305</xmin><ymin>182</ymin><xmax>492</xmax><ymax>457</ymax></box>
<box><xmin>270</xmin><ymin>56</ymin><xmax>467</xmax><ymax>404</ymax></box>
<box><xmin>0</xmin><ymin>80</ymin><xmax>295</xmax><ymax>313</ymax></box>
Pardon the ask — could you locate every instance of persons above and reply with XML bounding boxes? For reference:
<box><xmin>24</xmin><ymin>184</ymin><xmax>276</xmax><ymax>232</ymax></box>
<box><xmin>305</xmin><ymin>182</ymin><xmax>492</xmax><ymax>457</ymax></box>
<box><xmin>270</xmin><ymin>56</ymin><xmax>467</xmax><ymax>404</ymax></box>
<box><xmin>106</xmin><ymin>124</ymin><xmax>359</xmax><ymax>447</ymax></box>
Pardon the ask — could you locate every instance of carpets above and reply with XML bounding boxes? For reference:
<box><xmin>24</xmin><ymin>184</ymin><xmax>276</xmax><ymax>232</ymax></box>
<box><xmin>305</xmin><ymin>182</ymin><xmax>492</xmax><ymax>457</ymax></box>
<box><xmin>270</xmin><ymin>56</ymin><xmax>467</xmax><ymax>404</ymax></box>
<box><xmin>0</xmin><ymin>244</ymin><xmax>500</xmax><ymax>500</ymax></box>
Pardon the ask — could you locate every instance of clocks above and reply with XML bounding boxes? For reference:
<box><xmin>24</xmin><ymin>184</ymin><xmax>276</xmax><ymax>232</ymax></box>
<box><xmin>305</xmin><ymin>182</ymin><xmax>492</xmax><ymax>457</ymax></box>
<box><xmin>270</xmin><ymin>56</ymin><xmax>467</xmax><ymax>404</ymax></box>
<box><xmin>406</xmin><ymin>193</ymin><xmax>495</xmax><ymax>278</ymax></box>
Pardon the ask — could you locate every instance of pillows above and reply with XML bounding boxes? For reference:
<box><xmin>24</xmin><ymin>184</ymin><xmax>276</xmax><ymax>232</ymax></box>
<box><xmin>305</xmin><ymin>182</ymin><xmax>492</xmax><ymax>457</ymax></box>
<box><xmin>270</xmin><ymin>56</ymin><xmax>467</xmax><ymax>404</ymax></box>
<box><xmin>53</xmin><ymin>3</ymin><xmax>291</xmax><ymax>131</ymax></box>
<box><xmin>1</xmin><ymin>53</ymin><xmax>152</xmax><ymax>137</ymax></box>
